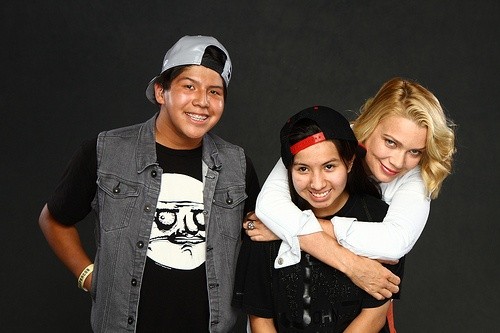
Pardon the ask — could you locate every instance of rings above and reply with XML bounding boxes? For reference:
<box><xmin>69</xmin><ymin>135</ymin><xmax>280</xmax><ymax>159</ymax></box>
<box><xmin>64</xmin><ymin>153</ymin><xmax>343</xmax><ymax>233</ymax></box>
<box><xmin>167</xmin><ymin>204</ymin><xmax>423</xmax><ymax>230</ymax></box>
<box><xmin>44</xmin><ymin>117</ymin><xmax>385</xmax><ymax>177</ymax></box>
<box><xmin>248</xmin><ymin>220</ymin><xmax>255</xmax><ymax>229</ymax></box>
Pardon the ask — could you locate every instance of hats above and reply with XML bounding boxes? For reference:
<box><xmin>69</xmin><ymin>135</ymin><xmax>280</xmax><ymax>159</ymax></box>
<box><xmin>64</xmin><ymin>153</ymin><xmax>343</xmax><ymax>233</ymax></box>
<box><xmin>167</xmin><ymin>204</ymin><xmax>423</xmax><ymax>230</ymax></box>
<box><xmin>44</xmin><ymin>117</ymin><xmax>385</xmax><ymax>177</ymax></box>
<box><xmin>280</xmin><ymin>106</ymin><xmax>366</xmax><ymax>169</ymax></box>
<box><xmin>146</xmin><ymin>35</ymin><xmax>233</xmax><ymax>106</ymax></box>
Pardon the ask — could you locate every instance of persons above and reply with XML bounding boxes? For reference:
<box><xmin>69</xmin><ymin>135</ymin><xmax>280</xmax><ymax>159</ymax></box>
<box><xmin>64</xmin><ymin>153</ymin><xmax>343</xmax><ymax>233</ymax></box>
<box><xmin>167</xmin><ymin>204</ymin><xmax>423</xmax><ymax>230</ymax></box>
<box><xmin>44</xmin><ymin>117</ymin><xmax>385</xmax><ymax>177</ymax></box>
<box><xmin>41</xmin><ymin>30</ymin><xmax>261</xmax><ymax>332</ymax></box>
<box><xmin>231</xmin><ymin>107</ymin><xmax>406</xmax><ymax>332</ymax></box>
<box><xmin>243</xmin><ymin>75</ymin><xmax>455</xmax><ymax>332</ymax></box>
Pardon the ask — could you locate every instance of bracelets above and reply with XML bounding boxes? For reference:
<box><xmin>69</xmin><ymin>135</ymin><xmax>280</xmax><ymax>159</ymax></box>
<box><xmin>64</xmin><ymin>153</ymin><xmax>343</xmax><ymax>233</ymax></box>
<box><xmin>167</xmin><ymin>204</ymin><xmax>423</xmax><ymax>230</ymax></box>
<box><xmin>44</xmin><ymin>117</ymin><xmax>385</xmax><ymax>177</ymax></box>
<box><xmin>77</xmin><ymin>263</ymin><xmax>94</xmax><ymax>292</ymax></box>
